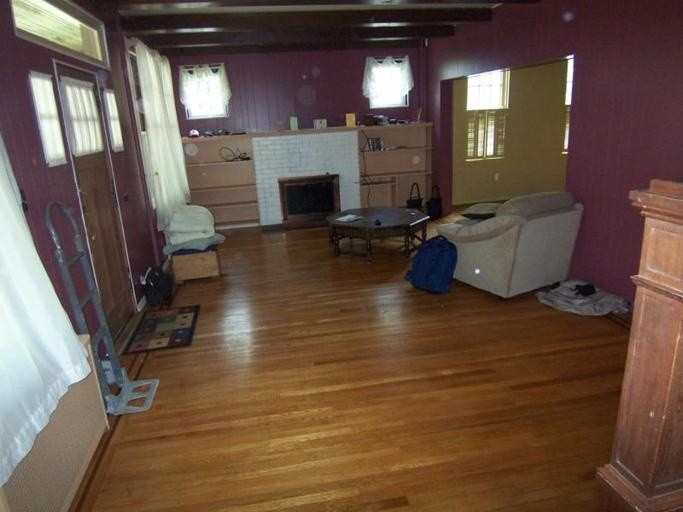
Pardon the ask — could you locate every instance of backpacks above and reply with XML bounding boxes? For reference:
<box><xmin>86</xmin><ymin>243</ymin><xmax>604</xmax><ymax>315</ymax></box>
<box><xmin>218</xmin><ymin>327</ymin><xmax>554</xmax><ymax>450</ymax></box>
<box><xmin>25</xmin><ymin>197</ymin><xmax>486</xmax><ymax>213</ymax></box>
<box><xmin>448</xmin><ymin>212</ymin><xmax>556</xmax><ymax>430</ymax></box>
<box><xmin>406</xmin><ymin>235</ymin><xmax>458</xmax><ymax>294</ymax></box>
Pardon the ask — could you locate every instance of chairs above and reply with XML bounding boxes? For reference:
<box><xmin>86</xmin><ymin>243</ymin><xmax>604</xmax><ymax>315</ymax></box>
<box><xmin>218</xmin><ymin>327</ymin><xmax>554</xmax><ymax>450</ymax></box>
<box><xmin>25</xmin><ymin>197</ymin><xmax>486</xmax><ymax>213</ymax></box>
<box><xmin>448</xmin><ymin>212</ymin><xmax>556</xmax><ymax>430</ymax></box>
<box><xmin>437</xmin><ymin>190</ymin><xmax>584</xmax><ymax>300</ymax></box>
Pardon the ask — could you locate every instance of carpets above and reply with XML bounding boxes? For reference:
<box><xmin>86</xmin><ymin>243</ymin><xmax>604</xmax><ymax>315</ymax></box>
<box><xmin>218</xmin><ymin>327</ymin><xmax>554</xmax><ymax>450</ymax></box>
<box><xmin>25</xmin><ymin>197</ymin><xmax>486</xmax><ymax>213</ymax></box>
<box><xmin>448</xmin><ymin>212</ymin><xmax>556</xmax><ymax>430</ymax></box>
<box><xmin>122</xmin><ymin>305</ymin><xmax>201</xmax><ymax>356</ymax></box>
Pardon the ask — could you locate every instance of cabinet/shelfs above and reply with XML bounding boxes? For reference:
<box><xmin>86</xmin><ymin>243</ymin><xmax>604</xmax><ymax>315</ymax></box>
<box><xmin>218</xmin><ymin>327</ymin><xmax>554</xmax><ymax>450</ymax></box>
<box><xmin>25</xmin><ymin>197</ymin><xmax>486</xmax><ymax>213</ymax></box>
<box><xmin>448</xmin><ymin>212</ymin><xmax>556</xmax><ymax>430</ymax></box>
<box><xmin>179</xmin><ymin>135</ymin><xmax>259</xmax><ymax>235</ymax></box>
<box><xmin>357</xmin><ymin>121</ymin><xmax>436</xmax><ymax>208</ymax></box>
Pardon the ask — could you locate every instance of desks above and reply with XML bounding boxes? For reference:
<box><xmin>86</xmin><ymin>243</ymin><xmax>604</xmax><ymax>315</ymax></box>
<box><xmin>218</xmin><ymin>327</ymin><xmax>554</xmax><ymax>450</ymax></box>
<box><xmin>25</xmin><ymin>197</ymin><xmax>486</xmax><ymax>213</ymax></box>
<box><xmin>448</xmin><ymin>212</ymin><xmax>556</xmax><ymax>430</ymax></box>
<box><xmin>325</xmin><ymin>208</ymin><xmax>431</xmax><ymax>264</ymax></box>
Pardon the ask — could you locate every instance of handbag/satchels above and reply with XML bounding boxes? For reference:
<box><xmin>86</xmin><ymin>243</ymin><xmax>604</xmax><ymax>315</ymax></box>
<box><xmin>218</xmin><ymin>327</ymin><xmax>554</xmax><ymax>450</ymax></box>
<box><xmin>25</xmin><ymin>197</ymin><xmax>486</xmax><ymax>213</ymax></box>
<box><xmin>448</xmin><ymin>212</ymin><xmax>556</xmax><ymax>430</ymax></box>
<box><xmin>405</xmin><ymin>197</ymin><xmax>422</xmax><ymax>211</ymax></box>
<box><xmin>428</xmin><ymin>197</ymin><xmax>443</xmax><ymax>219</ymax></box>
<box><xmin>141</xmin><ymin>266</ymin><xmax>172</xmax><ymax>305</ymax></box>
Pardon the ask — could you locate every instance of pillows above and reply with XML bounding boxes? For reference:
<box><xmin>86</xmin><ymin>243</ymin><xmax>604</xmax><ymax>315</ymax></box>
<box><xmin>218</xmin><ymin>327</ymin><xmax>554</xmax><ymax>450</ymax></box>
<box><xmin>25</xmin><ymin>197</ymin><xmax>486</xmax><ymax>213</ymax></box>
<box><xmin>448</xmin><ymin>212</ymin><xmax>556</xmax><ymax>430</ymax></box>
<box><xmin>455</xmin><ymin>202</ymin><xmax>503</xmax><ymax>227</ymax></box>
